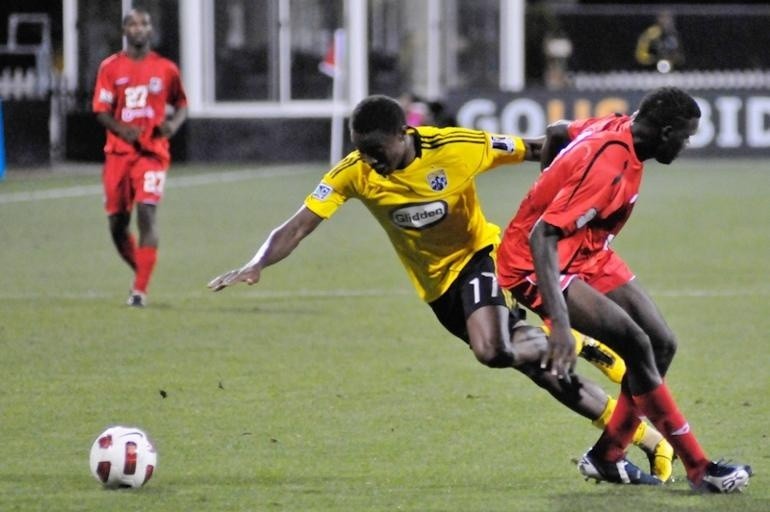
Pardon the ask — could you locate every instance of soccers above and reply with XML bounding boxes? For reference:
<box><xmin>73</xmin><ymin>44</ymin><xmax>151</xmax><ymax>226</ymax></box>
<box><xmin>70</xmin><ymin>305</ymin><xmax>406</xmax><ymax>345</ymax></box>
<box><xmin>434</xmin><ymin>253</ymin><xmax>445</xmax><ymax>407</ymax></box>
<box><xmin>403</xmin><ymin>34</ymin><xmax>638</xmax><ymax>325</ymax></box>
<box><xmin>90</xmin><ymin>427</ymin><xmax>156</xmax><ymax>490</ymax></box>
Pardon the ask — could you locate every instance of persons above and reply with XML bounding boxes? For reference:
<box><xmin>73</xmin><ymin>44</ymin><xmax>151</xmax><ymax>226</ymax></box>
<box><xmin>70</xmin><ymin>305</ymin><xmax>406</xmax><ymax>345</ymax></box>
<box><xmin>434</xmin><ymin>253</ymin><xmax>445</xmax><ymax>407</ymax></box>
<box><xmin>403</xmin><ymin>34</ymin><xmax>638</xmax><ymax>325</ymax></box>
<box><xmin>90</xmin><ymin>5</ymin><xmax>189</xmax><ymax>306</ymax></box>
<box><xmin>495</xmin><ymin>86</ymin><xmax>754</xmax><ymax>493</ymax></box>
<box><xmin>208</xmin><ymin>91</ymin><xmax>681</xmax><ymax>486</ymax></box>
<box><xmin>634</xmin><ymin>8</ymin><xmax>689</xmax><ymax>71</ymax></box>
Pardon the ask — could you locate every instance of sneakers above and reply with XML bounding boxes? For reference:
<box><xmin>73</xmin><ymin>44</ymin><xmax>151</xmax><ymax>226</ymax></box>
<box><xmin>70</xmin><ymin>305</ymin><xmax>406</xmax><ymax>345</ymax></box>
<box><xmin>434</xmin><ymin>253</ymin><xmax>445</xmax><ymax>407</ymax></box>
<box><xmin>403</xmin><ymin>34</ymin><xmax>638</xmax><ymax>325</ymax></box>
<box><xmin>576</xmin><ymin>336</ymin><xmax>627</xmax><ymax>384</ymax></box>
<box><xmin>687</xmin><ymin>459</ymin><xmax>752</xmax><ymax>495</ymax></box>
<box><xmin>577</xmin><ymin>447</ymin><xmax>664</xmax><ymax>485</ymax></box>
<box><xmin>127</xmin><ymin>291</ymin><xmax>145</xmax><ymax>306</ymax></box>
<box><xmin>647</xmin><ymin>437</ymin><xmax>677</xmax><ymax>484</ymax></box>
